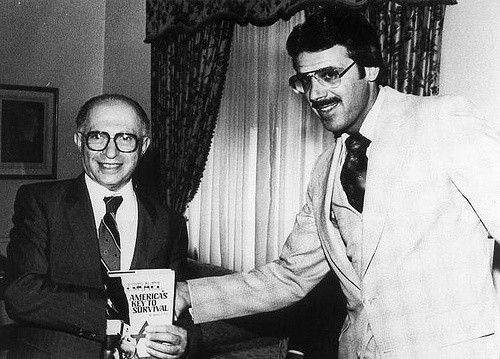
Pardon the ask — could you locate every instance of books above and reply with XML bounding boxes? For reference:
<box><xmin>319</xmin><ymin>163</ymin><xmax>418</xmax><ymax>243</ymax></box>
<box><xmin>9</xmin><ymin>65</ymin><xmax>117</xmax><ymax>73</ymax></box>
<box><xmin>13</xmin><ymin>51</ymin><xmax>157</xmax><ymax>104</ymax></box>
<box><xmin>104</xmin><ymin>268</ymin><xmax>176</xmax><ymax>358</ymax></box>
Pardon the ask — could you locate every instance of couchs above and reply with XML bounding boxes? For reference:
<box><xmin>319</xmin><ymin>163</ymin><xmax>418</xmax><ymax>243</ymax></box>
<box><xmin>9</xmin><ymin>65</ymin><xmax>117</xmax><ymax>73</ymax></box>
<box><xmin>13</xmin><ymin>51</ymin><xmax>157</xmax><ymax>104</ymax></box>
<box><xmin>176</xmin><ymin>258</ymin><xmax>291</xmax><ymax>359</ymax></box>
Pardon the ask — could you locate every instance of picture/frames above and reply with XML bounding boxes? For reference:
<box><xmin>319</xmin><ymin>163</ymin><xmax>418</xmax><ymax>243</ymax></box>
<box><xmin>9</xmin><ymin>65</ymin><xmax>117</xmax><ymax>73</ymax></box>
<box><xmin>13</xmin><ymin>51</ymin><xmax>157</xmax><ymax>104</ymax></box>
<box><xmin>0</xmin><ymin>83</ymin><xmax>58</xmax><ymax>181</ymax></box>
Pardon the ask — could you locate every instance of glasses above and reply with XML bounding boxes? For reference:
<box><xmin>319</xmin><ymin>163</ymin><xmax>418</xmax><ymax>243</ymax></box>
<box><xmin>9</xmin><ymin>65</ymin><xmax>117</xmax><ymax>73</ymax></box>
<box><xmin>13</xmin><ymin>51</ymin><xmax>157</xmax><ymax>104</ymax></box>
<box><xmin>77</xmin><ymin>131</ymin><xmax>144</xmax><ymax>153</ymax></box>
<box><xmin>288</xmin><ymin>62</ymin><xmax>359</xmax><ymax>95</ymax></box>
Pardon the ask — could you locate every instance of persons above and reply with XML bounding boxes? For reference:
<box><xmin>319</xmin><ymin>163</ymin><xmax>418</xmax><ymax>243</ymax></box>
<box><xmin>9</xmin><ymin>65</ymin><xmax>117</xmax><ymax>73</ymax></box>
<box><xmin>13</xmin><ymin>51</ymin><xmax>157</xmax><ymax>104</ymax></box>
<box><xmin>174</xmin><ymin>9</ymin><xmax>499</xmax><ymax>358</ymax></box>
<box><xmin>0</xmin><ymin>94</ymin><xmax>189</xmax><ymax>358</ymax></box>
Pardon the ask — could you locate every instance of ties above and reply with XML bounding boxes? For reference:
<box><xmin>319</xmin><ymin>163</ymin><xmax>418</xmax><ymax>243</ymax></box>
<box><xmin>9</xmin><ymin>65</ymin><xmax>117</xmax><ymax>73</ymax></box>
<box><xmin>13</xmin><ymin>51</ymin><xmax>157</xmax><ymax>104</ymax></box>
<box><xmin>340</xmin><ymin>131</ymin><xmax>371</xmax><ymax>214</ymax></box>
<box><xmin>97</xmin><ymin>196</ymin><xmax>129</xmax><ymax>322</ymax></box>
